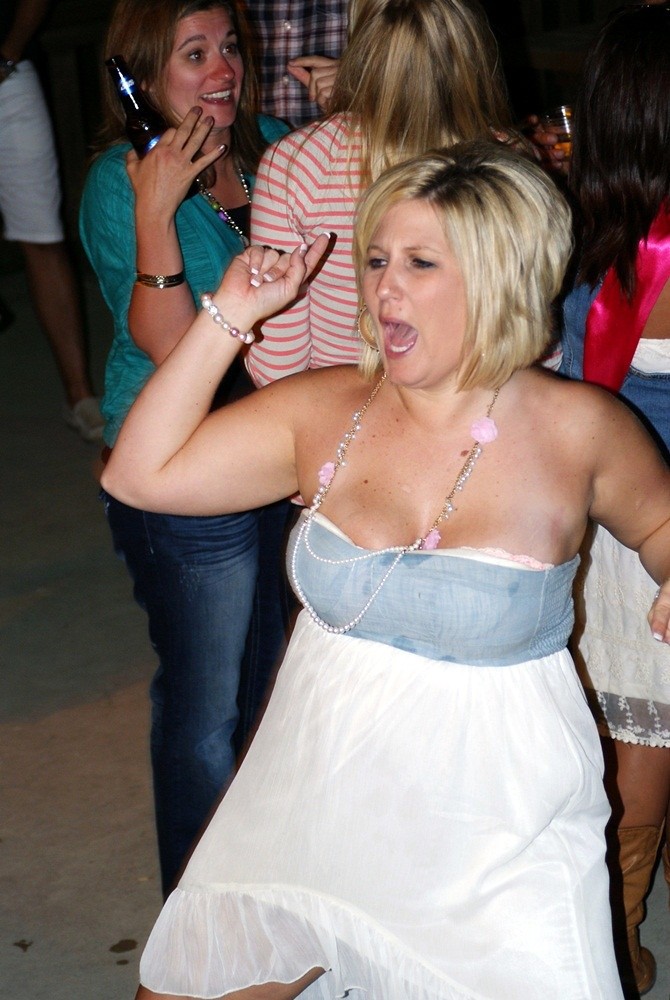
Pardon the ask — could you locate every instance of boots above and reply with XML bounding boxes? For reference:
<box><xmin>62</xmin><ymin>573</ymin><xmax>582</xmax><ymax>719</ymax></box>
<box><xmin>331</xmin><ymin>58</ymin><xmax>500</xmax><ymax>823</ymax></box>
<box><xmin>609</xmin><ymin>824</ymin><xmax>666</xmax><ymax>997</ymax></box>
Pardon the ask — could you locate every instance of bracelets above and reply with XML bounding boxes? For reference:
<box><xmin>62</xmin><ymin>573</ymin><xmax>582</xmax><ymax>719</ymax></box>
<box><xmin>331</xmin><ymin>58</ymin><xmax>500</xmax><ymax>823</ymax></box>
<box><xmin>199</xmin><ymin>291</ymin><xmax>255</xmax><ymax>344</ymax></box>
<box><xmin>136</xmin><ymin>270</ymin><xmax>184</xmax><ymax>289</ymax></box>
<box><xmin>0</xmin><ymin>53</ymin><xmax>15</xmax><ymax>74</ymax></box>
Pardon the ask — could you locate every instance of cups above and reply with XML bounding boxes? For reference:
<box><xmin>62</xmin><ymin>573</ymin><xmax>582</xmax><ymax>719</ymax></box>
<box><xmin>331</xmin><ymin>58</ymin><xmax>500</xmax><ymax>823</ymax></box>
<box><xmin>540</xmin><ymin>103</ymin><xmax>578</xmax><ymax>179</ymax></box>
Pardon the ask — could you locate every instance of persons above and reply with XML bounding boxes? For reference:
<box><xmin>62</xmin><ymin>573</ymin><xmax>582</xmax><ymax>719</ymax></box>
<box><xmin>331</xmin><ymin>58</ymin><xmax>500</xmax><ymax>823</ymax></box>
<box><xmin>97</xmin><ymin>141</ymin><xmax>670</xmax><ymax>1000</ymax></box>
<box><xmin>0</xmin><ymin>0</ymin><xmax>106</xmax><ymax>444</ymax></box>
<box><xmin>523</xmin><ymin>0</ymin><xmax>670</xmax><ymax>1000</ymax></box>
<box><xmin>78</xmin><ymin>0</ymin><xmax>309</xmax><ymax>893</ymax></box>
<box><xmin>242</xmin><ymin>0</ymin><xmax>352</xmax><ymax>129</ymax></box>
<box><xmin>244</xmin><ymin>0</ymin><xmax>545</xmax><ymax>633</ymax></box>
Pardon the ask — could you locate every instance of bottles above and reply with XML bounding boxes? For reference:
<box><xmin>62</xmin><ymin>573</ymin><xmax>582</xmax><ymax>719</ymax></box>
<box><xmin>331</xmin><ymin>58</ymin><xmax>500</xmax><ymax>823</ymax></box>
<box><xmin>105</xmin><ymin>54</ymin><xmax>207</xmax><ymax>202</ymax></box>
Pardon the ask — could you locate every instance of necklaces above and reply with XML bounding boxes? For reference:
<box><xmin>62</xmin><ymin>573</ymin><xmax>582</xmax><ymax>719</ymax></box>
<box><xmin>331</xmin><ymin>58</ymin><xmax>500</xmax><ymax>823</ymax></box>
<box><xmin>196</xmin><ymin>151</ymin><xmax>253</xmax><ymax>248</ymax></box>
<box><xmin>290</xmin><ymin>378</ymin><xmax>501</xmax><ymax>635</ymax></box>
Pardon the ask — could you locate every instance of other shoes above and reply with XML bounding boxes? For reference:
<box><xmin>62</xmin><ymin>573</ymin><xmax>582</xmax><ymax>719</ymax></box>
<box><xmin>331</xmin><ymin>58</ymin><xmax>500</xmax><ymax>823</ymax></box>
<box><xmin>60</xmin><ymin>396</ymin><xmax>105</xmax><ymax>441</ymax></box>
